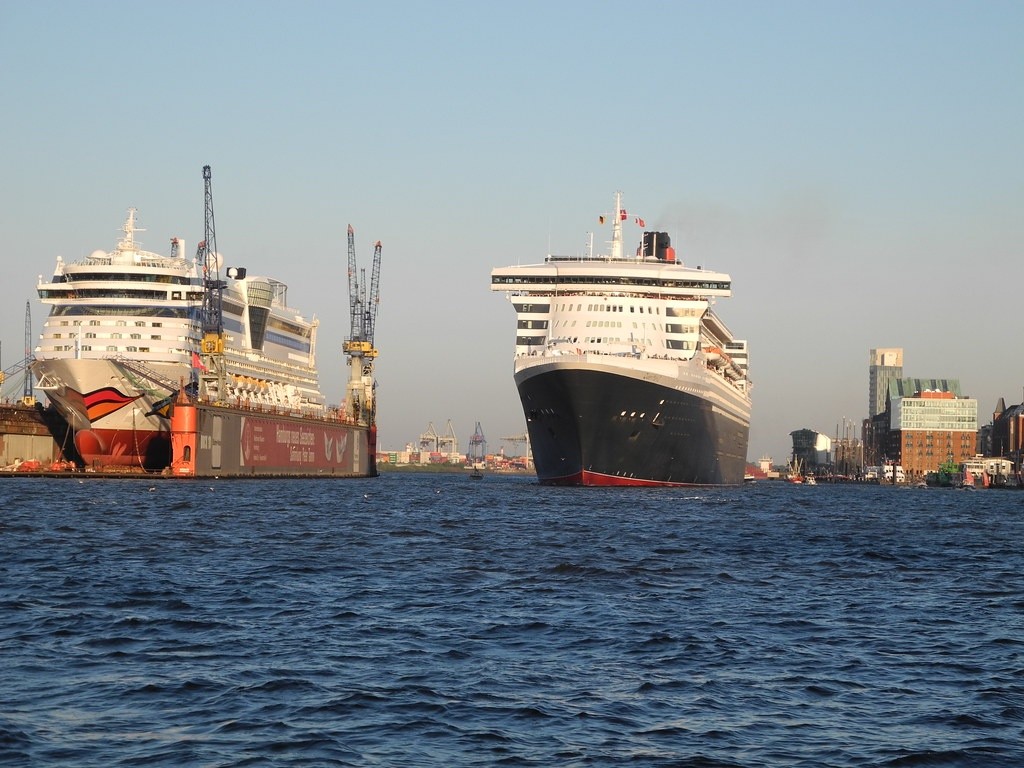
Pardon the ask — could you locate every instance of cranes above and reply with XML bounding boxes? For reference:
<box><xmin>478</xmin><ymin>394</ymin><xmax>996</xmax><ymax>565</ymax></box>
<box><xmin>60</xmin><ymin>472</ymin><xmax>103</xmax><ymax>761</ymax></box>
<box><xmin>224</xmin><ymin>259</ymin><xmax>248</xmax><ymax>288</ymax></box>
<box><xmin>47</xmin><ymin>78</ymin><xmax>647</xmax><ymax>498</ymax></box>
<box><xmin>498</xmin><ymin>430</ymin><xmax>531</xmax><ymax>471</ymax></box>
<box><xmin>187</xmin><ymin>163</ymin><xmax>226</xmax><ymax>404</ymax></box>
<box><xmin>418</xmin><ymin>419</ymin><xmax>487</xmax><ymax>462</ymax></box>
<box><xmin>340</xmin><ymin>221</ymin><xmax>384</xmax><ymax>377</ymax></box>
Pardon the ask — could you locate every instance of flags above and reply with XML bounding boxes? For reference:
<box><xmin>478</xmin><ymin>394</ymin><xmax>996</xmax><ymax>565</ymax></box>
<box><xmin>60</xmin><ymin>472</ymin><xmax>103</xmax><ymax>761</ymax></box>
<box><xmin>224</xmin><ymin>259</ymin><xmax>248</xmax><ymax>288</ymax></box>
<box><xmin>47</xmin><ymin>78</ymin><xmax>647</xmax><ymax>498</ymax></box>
<box><xmin>600</xmin><ymin>216</ymin><xmax>603</xmax><ymax>224</ymax></box>
<box><xmin>193</xmin><ymin>353</ymin><xmax>206</xmax><ymax>372</ymax></box>
<box><xmin>620</xmin><ymin>210</ymin><xmax>626</xmax><ymax>220</ymax></box>
<box><xmin>635</xmin><ymin>218</ymin><xmax>644</xmax><ymax>227</ymax></box>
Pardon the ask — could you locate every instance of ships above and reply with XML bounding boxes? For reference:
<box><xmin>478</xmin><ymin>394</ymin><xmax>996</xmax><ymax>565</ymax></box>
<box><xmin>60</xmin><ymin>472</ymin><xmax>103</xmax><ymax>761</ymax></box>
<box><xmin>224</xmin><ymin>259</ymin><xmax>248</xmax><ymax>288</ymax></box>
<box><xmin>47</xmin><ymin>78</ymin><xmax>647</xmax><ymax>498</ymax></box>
<box><xmin>490</xmin><ymin>191</ymin><xmax>755</xmax><ymax>490</ymax></box>
<box><xmin>24</xmin><ymin>205</ymin><xmax>377</xmax><ymax>480</ymax></box>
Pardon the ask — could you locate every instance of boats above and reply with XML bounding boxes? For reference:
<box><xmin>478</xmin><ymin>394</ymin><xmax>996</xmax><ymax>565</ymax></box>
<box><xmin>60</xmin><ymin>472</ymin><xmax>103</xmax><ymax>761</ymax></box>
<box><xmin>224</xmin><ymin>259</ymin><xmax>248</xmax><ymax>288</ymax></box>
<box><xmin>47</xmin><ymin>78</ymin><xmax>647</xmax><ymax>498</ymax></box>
<box><xmin>805</xmin><ymin>472</ymin><xmax>817</xmax><ymax>486</ymax></box>
<box><xmin>230</xmin><ymin>374</ymin><xmax>269</xmax><ymax>395</ymax></box>
<box><xmin>744</xmin><ymin>473</ymin><xmax>755</xmax><ymax>481</ymax></box>
<box><xmin>704</xmin><ymin>345</ymin><xmax>730</xmax><ymax>367</ymax></box>
<box><xmin>783</xmin><ymin>454</ymin><xmax>804</xmax><ymax>483</ymax></box>
<box><xmin>470</xmin><ymin>471</ymin><xmax>484</xmax><ymax>480</ymax></box>
